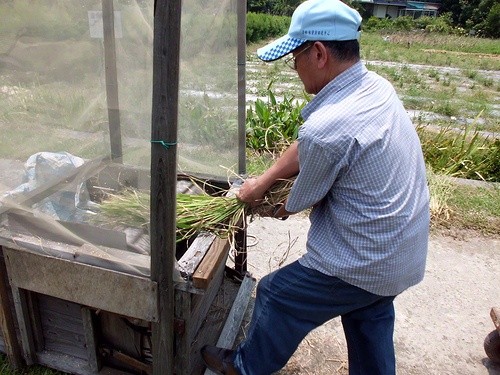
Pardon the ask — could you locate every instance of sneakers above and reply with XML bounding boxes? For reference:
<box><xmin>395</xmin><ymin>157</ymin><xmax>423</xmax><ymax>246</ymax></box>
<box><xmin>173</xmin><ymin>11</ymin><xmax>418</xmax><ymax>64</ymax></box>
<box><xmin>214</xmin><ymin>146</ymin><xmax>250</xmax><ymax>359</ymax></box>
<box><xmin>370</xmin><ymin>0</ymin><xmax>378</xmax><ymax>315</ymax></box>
<box><xmin>200</xmin><ymin>344</ymin><xmax>241</xmax><ymax>375</ymax></box>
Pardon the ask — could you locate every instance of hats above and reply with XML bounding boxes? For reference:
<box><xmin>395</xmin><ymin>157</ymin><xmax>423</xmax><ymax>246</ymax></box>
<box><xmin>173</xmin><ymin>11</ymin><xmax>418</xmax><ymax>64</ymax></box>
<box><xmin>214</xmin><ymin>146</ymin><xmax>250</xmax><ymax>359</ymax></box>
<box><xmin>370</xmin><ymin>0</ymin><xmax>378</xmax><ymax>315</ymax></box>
<box><xmin>256</xmin><ymin>0</ymin><xmax>362</xmax><ymax>62</ymax></box>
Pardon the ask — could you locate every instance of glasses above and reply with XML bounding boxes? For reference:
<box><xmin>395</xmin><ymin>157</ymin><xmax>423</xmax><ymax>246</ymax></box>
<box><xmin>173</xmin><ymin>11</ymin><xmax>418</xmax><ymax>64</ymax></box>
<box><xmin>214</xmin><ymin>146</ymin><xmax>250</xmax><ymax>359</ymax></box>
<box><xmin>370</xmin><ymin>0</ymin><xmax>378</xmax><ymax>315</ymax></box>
<box><xmin>284</xmin><ymin>45</ymin><xmax>311</xmax><ymax>69</ymax></box>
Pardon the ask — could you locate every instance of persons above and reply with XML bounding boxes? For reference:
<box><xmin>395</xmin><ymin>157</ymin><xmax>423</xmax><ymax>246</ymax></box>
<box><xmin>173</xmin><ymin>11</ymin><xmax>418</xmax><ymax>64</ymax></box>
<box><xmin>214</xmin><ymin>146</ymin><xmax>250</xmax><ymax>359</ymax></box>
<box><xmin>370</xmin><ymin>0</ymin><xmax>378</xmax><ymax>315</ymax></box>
<box><xmin>200</xmin><ymin>0</ymin><xmax>429</xmax><ymax>375</ymax></box>
